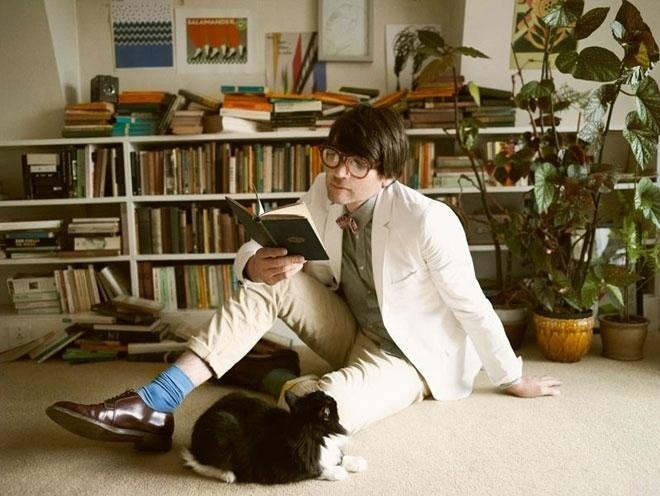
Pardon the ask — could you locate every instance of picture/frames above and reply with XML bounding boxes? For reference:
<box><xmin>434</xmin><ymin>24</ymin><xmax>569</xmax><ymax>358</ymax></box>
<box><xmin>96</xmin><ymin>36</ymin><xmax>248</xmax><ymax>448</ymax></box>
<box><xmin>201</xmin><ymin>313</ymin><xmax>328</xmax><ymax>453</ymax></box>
<box><xmin>316</xmin><ymin>0</ymin><xmax>374</xmax><ymax>62</ymax></box>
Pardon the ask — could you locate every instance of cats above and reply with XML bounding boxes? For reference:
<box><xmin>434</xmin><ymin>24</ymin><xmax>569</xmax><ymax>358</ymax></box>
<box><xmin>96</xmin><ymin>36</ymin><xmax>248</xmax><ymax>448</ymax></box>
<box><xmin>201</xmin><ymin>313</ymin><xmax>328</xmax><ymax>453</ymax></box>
<box><xmin>179</xmin><ymin>389</ymin><xmax>367</xmax><ymax>486</ymax></box>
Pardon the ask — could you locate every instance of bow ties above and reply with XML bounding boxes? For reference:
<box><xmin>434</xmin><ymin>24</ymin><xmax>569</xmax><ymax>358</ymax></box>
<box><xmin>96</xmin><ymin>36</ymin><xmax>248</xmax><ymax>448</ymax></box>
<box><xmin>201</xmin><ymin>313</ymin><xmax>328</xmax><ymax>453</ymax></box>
<box><xmin>336</xmin><ymin>215</ymin><xmax>359</xmax><ymax>232</ymax></box>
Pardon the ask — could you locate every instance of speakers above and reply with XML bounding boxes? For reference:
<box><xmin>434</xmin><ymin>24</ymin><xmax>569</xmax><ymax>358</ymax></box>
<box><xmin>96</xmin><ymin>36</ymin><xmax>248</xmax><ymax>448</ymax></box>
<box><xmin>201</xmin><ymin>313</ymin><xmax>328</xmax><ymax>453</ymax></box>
<box><xmin>90</xmin><ymin>74</ymin><xmax>120</xmax><ymax>103</ymax></box>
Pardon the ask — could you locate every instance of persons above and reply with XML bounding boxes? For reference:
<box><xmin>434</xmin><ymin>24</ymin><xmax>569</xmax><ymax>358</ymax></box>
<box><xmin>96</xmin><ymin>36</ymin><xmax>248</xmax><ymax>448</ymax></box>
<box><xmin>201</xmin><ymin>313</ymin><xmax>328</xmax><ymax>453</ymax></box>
<box><xmin>44</xmin><ymin>104</ymin><xmax>564</xmax><ymax>452</ymax></box>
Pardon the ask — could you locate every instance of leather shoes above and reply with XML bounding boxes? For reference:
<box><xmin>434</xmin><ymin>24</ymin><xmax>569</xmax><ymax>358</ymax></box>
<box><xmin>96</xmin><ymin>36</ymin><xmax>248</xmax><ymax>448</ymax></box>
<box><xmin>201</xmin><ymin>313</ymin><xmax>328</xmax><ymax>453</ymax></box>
<box><xmin>207</xmin><ymin>349</ymin><xmax>300</xmax><ymax>391</ymax></box>
<box><xmin>46</xmin><ymin>389</ymin><xmax>173</xmax><ymax>450</ymax></box>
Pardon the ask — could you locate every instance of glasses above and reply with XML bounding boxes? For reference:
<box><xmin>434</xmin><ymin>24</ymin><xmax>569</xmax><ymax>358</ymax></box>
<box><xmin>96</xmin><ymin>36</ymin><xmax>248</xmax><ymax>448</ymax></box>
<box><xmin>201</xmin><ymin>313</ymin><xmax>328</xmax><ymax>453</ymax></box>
<box><xmin>318</xmin><ymin>143</ymin><xmax>382</xmax><ymax>179</ymax></box>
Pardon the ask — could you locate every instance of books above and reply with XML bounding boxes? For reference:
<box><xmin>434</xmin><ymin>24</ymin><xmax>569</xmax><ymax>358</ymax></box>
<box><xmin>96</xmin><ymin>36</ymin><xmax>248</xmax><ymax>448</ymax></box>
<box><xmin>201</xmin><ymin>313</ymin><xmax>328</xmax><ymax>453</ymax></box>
<box><xmin>0</xmin><ymin>83</ymin><xmax>529</xmax><ymax>368</ymax></box>
